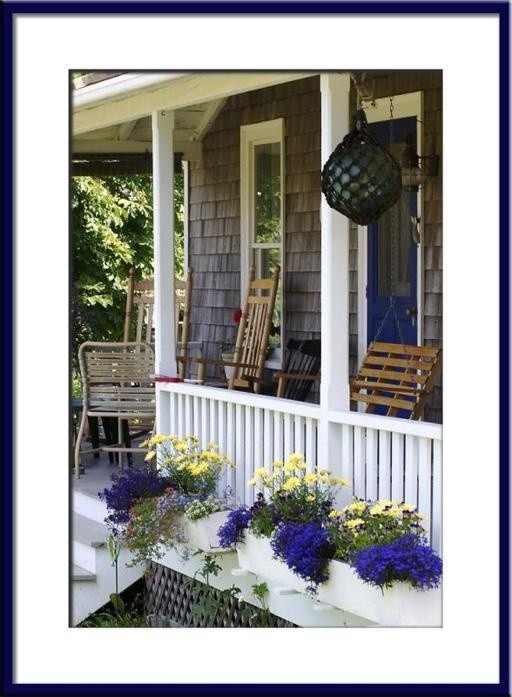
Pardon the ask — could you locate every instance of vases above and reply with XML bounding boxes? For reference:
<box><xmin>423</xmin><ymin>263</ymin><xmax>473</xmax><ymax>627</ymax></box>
<box><xmin>160</xmin><ymin>508</ymin><xmax>233</xmax><ymax>555</ymax></box>
<box><xmin>239</xmin><ymin>528</ymin><xmax>440</xmax><ymax>628</ymax></box>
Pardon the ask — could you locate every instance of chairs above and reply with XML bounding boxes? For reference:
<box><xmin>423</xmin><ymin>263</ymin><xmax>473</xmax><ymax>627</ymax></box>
<box><xmin>341</xmin><ymin>336</ymin><xmax>437</xmax><ymax>419</ymax></box>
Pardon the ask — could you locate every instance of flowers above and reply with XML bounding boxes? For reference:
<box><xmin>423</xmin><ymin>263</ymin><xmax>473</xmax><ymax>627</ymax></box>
<box><xmin>214</xmin><ymin>454</ymin><xmax>443</xmax><ymax>628</ymax></box>
<box><xmin>98</xmin><ymin>432</ymin><xmax>237</xmax><ymax>561</ymax></box>
